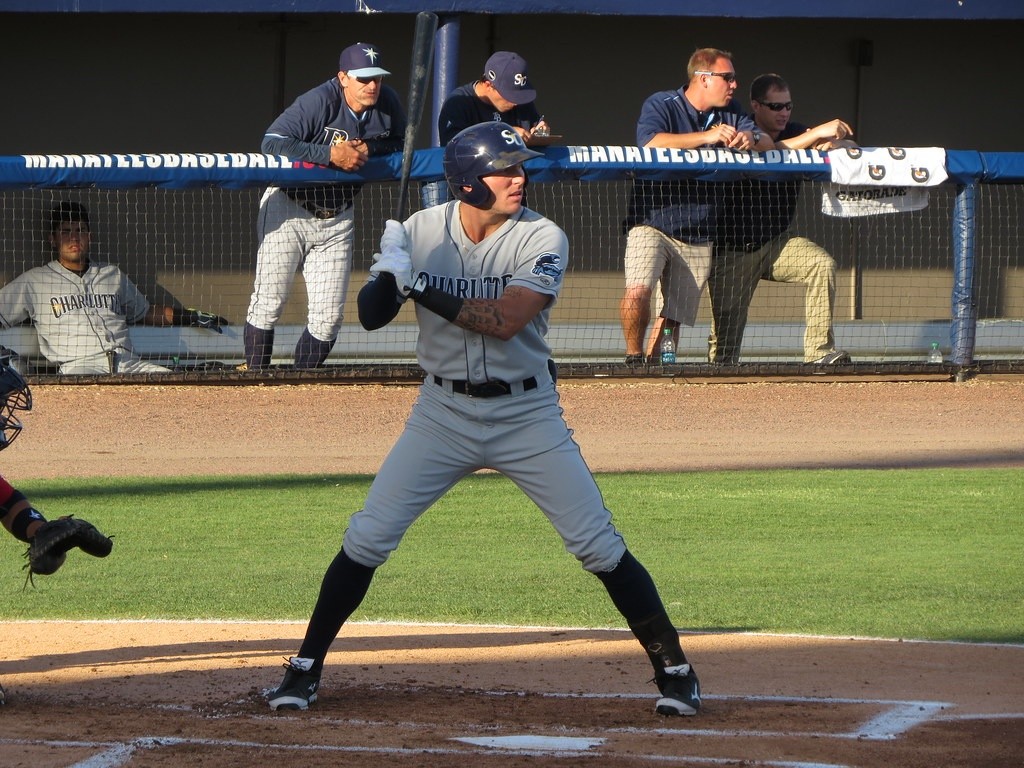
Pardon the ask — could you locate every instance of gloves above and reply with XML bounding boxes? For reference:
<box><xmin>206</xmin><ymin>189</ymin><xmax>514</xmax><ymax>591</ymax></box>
<box><xmin>370</xmin><ymin>244</ymin><xmax>431</xmax><ymax>302</ymax></box>
<box><xmin>173</xmin><ymin>306</ymin><xmax>228</xmax><ymax>334</ymax></box>
<box><xmin>380</xmin><ymin>219</ymin><xmax>407</xmax><ymax>252</ymax></box>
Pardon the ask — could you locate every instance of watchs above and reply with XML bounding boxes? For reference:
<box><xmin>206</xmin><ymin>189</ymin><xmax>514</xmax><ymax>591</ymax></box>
<box><xmin>752</xmin><ymin>131</ymin><xmax>760</xmax><ymax>145</ymax></box>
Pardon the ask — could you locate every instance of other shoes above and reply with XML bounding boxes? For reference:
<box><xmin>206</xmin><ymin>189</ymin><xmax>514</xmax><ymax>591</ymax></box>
<box><xmin>811</xmin><ymin>350</ymin><xmax>851</xmax><ymax>364</ymax></box>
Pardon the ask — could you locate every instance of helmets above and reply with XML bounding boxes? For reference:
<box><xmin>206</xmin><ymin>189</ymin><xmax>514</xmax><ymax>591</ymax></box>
<box><xmin>1</xmin><ymin>364</ymin><xmax>32</xmax><ymax>451</ymax></box>
<box><xmin>442</xmin><ymin>121</ymin><xmax>545</xmax><ymax>207</ymax></box>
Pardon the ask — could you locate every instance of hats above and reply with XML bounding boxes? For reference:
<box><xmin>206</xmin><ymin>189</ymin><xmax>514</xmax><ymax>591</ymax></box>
<box><xmin>339</xmin><ymin>42</ymin><xmax>392</xmax><ymax>77</ymax></box>
<box><xmin>483</xmin><ymin>50</ymin><xmax>536</xmax><ymax>104</ymax></box>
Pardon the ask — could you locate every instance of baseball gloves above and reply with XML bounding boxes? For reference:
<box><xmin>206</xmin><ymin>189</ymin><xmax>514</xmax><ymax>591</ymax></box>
<box><xmin>21</xmin><ymin>514</ymin><xmax>115</xmax><ymax>593</ymax></box>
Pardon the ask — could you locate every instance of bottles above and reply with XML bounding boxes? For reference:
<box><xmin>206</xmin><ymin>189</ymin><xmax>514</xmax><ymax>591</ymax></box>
<box><xmin>169</xmin><ymin>357</ymin><xmax>182</xmax><ymax>372</ymax></box>
<box><xmin>660</xmin><ymin>328</ymin><xmax>677</xmax><ymax>365</ymax></box>
<box><xmin>928</xmin><ymin>342</ymin><xmax>943</xmax><ymax>363</ymax></box>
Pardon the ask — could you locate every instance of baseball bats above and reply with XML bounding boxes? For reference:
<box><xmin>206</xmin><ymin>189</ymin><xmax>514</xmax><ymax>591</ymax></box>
<box><xmin>393</xmin><ymin>11</ymin><xmax>440</xmax><ymax>224</ymax></box>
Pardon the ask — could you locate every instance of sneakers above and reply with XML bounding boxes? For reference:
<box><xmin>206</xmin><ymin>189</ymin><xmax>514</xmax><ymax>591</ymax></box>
<box><xmin>646</xmin><ymin>664</ymin><xmax>702</xmax><ymax>716</ymax></box>
<box><xmin>268</xmin><ymin>656</ymin><xmax>323</xmax><ymax>710</ymax></box>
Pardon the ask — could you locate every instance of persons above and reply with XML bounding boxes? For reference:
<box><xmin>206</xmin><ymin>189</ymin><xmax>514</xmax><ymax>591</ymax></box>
<box><xmin>706</xmin><ymin>72</ymin><xmax>853</xmax><ymax>366</ymax></box>
<box><xmin>0</xmin><ymin>345</ymin><xmax>112</xmax><ymax>574</ymax></box>
<box><xmin>267</xmin><ymin>120</ymin><xmax>701</xmax><ymax>716</ymax></box>
<box><xmin>438</xmin><ymin>50</ymin><xmax>550</xmax><ymax>148</ymax></box>
<box><xmin>243</xmin><ymin>43</ymin><xmax>405</xmax><ymax>370</ymax></box>
<box><xmin>619</xmin><ymin>47</ymin><xmax>776</xmax><ymax>364</ymax></box>
<box><xmin>0</xmin><ymin>202</ymin><xmax>229</xmax><ymax>375</ymax></box>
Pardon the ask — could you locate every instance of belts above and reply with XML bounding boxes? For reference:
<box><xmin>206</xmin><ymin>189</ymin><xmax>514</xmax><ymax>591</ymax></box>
<box><xmin>434</xmin><ymin>375</ymin><xmax>538</xmax><ymax>398</ymax></box>
<box><xmin>281</xmin><ymin>187</ymin><xmax>353</xmax><ymax>219</ymax></box>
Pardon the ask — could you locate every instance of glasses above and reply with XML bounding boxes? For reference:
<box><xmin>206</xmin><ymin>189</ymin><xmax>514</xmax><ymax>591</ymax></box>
<box><xmin>760</xmin><ymin>102</ymin><xmax>793</xmax><ymax>111</ymax></box>
<box><xmin>356</xmin><ymin>77</ymin><xmax>384</xmax><ymax>84</ymax></box>
<box><xmin>695</xmin><ymin>71</ymin><xmax>736</xmax><ymax>83</ymax></box>
